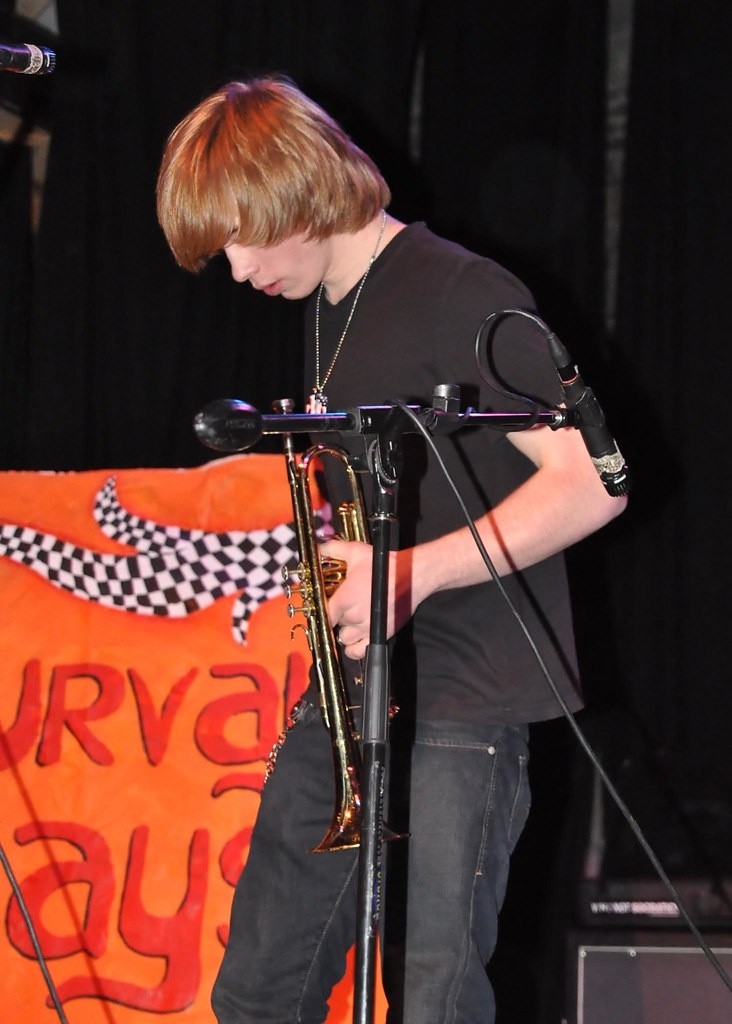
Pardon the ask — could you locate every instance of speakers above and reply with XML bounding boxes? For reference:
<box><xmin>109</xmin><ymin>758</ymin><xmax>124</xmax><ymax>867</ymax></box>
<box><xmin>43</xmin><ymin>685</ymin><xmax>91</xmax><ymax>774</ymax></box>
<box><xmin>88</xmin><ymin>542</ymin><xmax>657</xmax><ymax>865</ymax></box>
<box><xmin>566</xmin><ymin>931</ymin><xmax>732</xmax><ymax>1024</ymax></box>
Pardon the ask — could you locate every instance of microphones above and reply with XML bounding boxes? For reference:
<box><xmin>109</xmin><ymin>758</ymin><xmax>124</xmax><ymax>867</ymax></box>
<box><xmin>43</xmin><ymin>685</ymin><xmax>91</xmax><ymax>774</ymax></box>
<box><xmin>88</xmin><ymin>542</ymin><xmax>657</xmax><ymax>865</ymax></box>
<box><xmin>0</xmin><ymin>43</ymin><xmax>57</xmax><ymax>74</ymax></box>
<box><xmin>545</xmin><ymin>333</ymin><xmax>632</xmax><ymax>498</ymax></box>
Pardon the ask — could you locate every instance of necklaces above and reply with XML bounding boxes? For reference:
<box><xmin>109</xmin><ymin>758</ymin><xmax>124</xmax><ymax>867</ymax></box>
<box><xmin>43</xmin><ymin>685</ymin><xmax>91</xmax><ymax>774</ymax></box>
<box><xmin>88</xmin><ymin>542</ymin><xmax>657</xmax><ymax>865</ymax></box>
<box><xmin>304</xmin><ymin>208</ymin><xmax>385</xmax><ymax>413</ymax></box>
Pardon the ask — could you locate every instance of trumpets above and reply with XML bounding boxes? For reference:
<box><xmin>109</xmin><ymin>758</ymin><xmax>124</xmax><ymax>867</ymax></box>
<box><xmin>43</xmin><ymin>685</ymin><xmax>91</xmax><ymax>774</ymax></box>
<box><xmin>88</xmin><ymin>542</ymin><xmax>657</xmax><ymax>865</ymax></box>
<box><xmin>272</xmin><ymin>397</ymin><xmax>412</xmax><ymax>857</ymax></box>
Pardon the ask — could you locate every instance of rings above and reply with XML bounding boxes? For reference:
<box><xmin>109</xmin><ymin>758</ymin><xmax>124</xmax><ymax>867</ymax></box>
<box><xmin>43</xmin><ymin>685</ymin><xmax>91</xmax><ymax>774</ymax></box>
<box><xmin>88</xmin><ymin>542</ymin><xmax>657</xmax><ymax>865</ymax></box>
<box><xmin>337</xmin><ymin>627</ymin><xmax>346</xmax><ymax>645</ymax></box>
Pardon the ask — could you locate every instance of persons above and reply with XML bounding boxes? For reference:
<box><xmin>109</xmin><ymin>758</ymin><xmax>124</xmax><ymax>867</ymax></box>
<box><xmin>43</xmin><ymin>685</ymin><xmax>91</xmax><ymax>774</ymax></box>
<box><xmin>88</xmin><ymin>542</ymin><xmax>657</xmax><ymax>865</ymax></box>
<box><xmin>153</xmin><ymin>84</ymin><xmax>626</xmax><ymax>1024</ymax></box>
